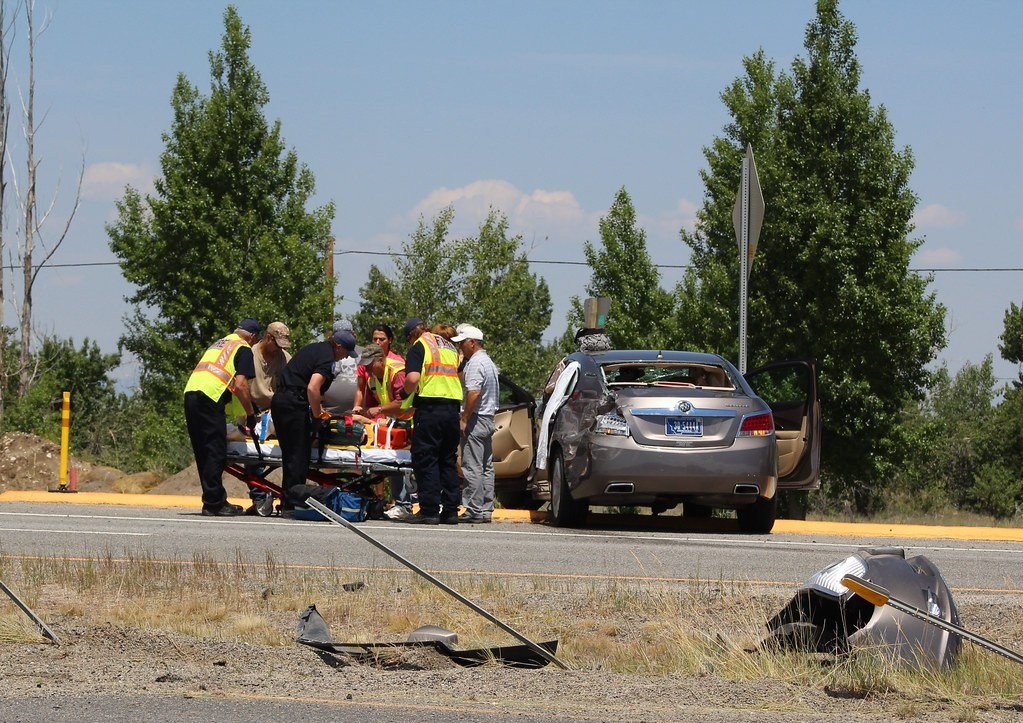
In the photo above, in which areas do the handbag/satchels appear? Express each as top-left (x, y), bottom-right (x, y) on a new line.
top-left (292, 483), bottom-right (369, 522)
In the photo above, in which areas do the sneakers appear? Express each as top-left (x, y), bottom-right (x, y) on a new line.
top-left (456, 513), bottom-right (491, 524)
top-left (438, 512), bottom-right (458, 524)
top-left (383, 504), bottom-right (413, 521)
top-left (403, 508), bottom-right (440, 525)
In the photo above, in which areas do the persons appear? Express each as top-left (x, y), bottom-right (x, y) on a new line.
top-left (618, 369), bottom-right (640, 382)
top-left (183, 317), bottom-right (500, 525)
top-left (690, 368), bottom-right (708, 386)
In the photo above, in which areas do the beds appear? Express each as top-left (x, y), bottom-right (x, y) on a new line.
top-left (226, 441), bottom-right (414, 517)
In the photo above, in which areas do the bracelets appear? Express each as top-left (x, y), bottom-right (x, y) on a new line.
top-left (379, 407), bottom-right (382, 414)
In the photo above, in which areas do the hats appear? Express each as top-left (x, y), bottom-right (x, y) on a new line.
top-left (266, 322), bottom-right (292, 348)
top-left (236, 318), bottom-right (261, 336)
top-left (332, 320), bottom-right (353, 333)
top-left (456, 323), bottom-right (472, 333)
top-left (450, 326), bottom-right (484, 342)
top-left (333, 331), bottom-right (358, 359)
top-left (357, 344), bottom-right (385, 366)
top-left (405, 318), bottom-right (423, 339)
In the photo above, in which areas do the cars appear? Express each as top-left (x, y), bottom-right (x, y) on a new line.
top-left (487, 348), bottom-right (822, 534)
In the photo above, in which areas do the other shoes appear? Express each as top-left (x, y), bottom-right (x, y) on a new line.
top-left (202, 503), bottom-right (243, 516)
